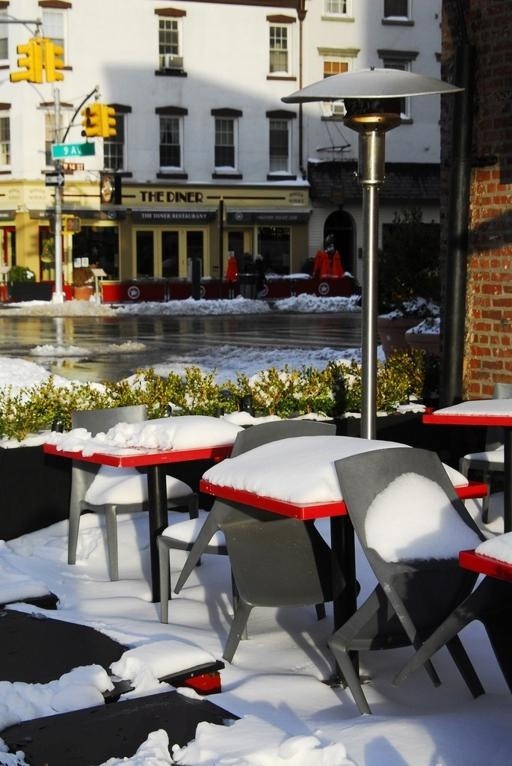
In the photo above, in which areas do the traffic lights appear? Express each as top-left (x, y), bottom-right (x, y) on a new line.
top-left (42, 38), bottom-right (64, 84)
top-left (81, 102), bottom-right (116, 139)
top-left (10, 37), bottom-right (42, 85)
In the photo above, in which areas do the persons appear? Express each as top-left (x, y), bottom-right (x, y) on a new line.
top-left (254, 255), bottom-right (268, 300)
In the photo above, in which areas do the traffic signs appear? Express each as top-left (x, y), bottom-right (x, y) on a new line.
top-left (45, 141), bottom-right (95, 186)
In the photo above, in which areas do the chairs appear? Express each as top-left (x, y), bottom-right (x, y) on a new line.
top-left (156, 419), bottom-right (512, 715)
top-left (460, 382), bottom-right (512, 523)
top-left (68, 406), bottom-right (205, 581)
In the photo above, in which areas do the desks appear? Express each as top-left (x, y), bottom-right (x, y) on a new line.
top-left (0, 591), bottom-right (240, 766)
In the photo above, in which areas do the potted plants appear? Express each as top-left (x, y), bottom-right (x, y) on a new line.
top-left (8, 267), bottom-right (38, 301)
top-left (73, 267), bottom-right (96, 300)
